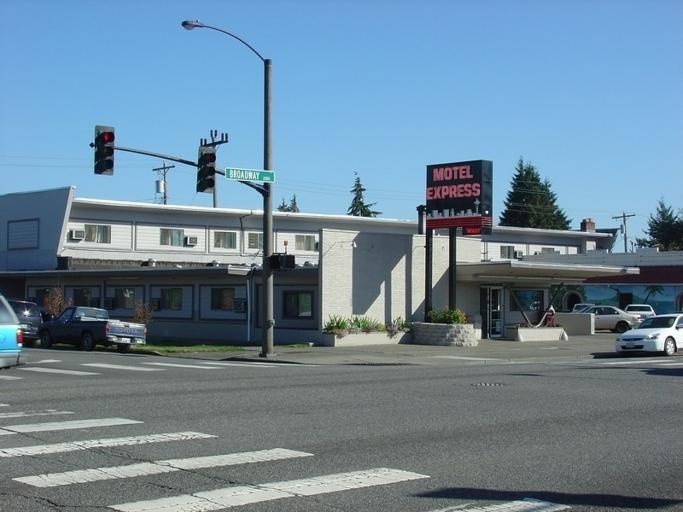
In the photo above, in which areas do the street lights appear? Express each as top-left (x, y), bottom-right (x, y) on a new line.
top-left (178, 19), bottom-right (277, 355)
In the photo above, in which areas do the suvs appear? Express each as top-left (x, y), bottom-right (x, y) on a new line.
top-left (0, 294), bottom-right (144, 368)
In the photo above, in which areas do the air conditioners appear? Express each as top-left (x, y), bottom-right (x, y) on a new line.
top-left (69, 228), bottom-right (85, 240)
top-left (183, 235), bottom-right (197, 246)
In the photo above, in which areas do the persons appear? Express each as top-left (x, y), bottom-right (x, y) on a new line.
top-left (545, 304), bottom-right (556, 327)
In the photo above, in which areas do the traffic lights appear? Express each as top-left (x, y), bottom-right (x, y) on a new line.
top-left (269, 254), bottom-right (294, 269)
top-left (195, 146), bottom-right (215, 195)
top-left (94, 125), bottom-right (113, 176)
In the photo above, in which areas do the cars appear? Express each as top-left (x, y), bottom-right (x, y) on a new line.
top-left (527, 300), bottom-right (539, 310)
top-left (570, 302), bottom-right (656, 333)
top-left (614, 313), bottom-right (682, 355)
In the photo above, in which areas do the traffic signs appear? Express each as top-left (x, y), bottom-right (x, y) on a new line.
top-left (224, 167), bottom-right (275, 184)
